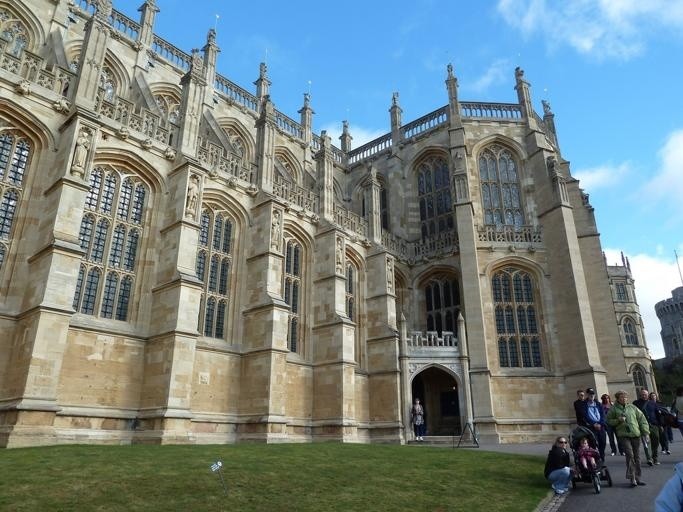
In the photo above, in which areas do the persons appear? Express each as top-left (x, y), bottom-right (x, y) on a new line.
top-left (410, 397), bottom-right (425, 440)
top-left (543, 435), bottom-right (576, 494)
top-left (575, 436), bottom-right (599, 469)
top-left (648, 391), bottom-right (671, 455)
top-left (631, 389), bottom-right (665, 466)
top-left (605, 390), bottom-right (651, 486)
top-left (582, 387), bottom-right (606, 462)
top-left (386, 261), bottom-right (393, 288)
top-left (272, 213), bottom-right (279, 241)
top-left (335, 239), bottom-right (343, 267)
top-left (667, 385), bottom-right (683, 443)
top-left (573, 389), bottom-right (587, 426)
top-left (185, 178), bottom-right (198, 213)
top-left (74, 132), bottom-right (90, 168)
top-left (599, 394), bottom-right (625, 457)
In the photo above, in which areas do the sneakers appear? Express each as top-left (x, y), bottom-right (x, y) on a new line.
top-left (552, 483), bottom-right (569, 495)
top-left (415, 436), bottom-right (423, 441)
top-left (610, 450), bottom-right (672, 487)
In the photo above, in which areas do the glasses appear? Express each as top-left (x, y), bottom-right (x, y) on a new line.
top-left (558, 442), bottom-right (567, 444)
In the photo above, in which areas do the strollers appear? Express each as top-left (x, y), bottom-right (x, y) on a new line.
top-left (567, 426), bottom-right (613, 495)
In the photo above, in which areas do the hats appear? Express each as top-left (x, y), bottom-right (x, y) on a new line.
top-left (586, 388), bottom-right (596, 394)
